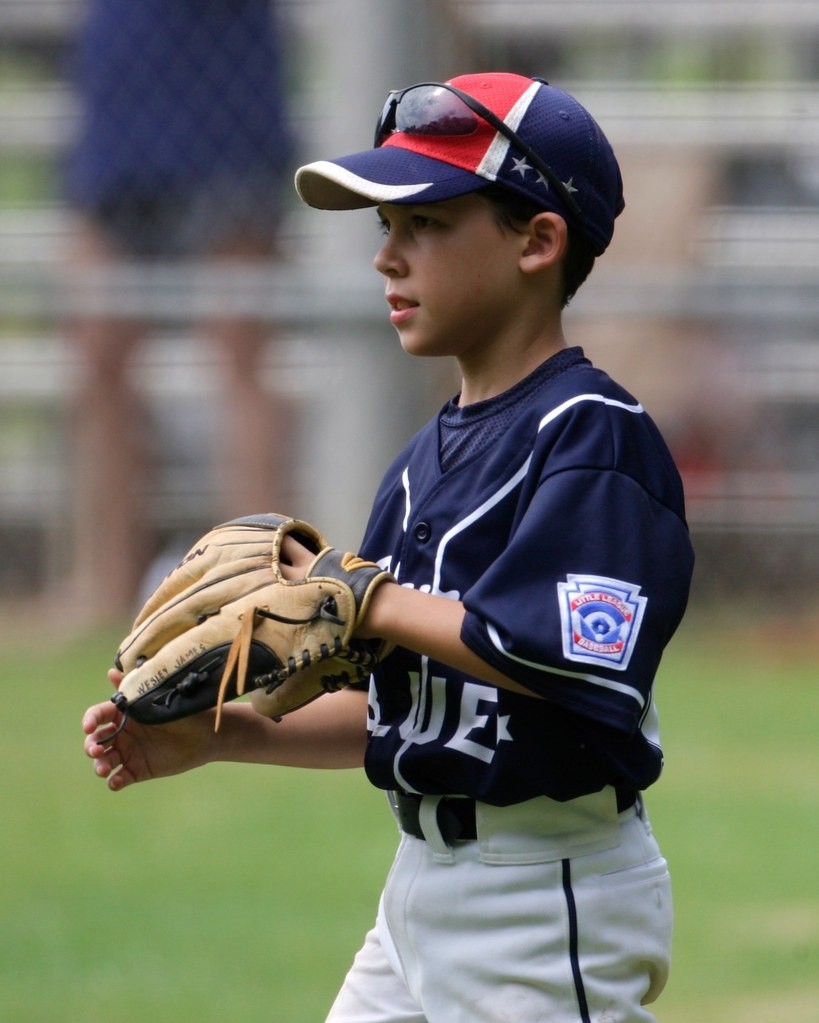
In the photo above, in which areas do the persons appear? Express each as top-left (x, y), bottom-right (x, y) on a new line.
top-left (34, 0), bottom-right (304, 669)
top-left (82, 70), bottom-right (694, 1022)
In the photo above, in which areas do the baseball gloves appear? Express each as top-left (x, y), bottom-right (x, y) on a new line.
top-left (113, 512), bottom-right (399, 729)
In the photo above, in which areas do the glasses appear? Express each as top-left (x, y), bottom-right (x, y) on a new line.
top-left (373, 81), bottom-right (581, 218)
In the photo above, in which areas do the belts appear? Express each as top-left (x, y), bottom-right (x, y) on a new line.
top-left (385, 789), bottom-right (636, 842)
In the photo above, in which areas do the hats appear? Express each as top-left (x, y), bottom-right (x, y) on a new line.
top-left (294, 71), bottom-right (625, 254)
